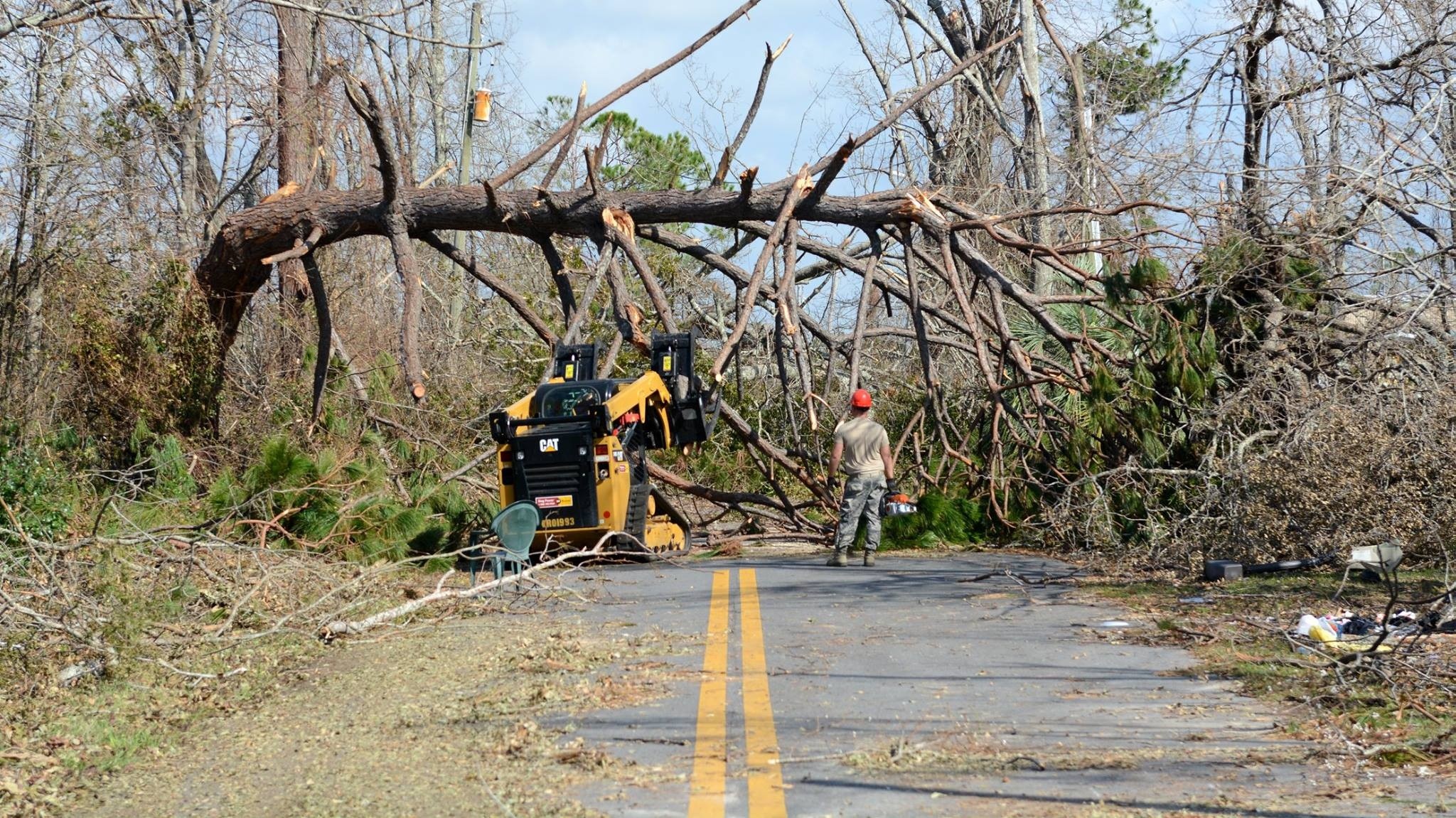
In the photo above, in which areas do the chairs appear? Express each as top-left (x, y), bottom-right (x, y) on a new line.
top-left (469, 499), bottom-right (541, 591)
top-left (1335, 528), bottom-right (1404, 602)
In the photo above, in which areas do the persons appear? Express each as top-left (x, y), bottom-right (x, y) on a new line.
top-left (827, 390), bottom-right (896, 567)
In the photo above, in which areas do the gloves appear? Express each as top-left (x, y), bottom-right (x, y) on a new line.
top-left (886, 479), bottom-right (901, 495)
top-left (828, 477), bottom-right (838, 492)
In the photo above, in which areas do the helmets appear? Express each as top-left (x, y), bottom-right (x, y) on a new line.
top-left (850, 389), bottom-right (871, 407)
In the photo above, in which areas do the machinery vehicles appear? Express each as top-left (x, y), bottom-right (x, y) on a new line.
top-left (490, 332), bottom-right (722, 564)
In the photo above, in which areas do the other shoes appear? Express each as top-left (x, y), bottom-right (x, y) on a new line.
top-left (865, 556), bottom-right (875, 566)
top-left (827, 553), bottom-right (847, 566)
